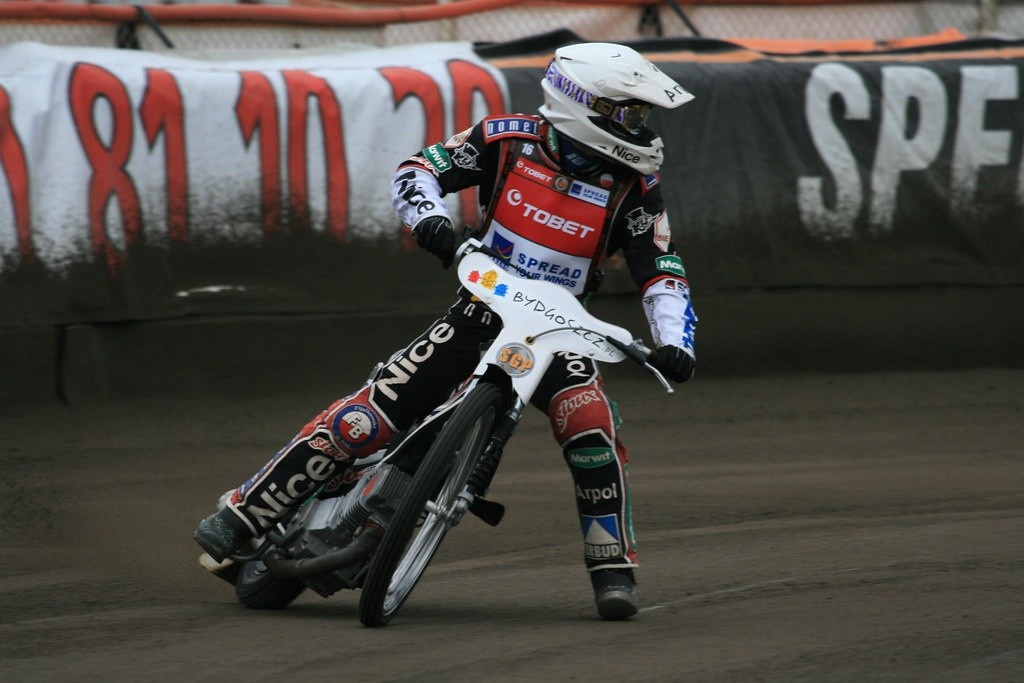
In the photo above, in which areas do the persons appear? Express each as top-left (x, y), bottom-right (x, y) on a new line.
top-left (192, 43), bottom-right (700, 619)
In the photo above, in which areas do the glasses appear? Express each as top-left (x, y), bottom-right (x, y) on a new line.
top-left (593, 98), bottom-right (657, 132)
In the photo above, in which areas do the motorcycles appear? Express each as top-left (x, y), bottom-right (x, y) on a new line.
top-left (199, 223), bottom-right (697, 629)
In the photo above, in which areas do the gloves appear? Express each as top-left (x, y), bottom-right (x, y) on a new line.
top-left (658, 346), bottom-right (692, 384)
top-left (415, 218), bottom-right (455, 268)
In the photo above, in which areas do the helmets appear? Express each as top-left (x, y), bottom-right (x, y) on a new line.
top-left (538, 42), bottom-right (696, 177)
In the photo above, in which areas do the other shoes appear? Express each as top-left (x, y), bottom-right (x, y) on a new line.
top-left (194, 512), bottom-right (231, 564)
top-left (595, 585), bottom-right (637, 621)
